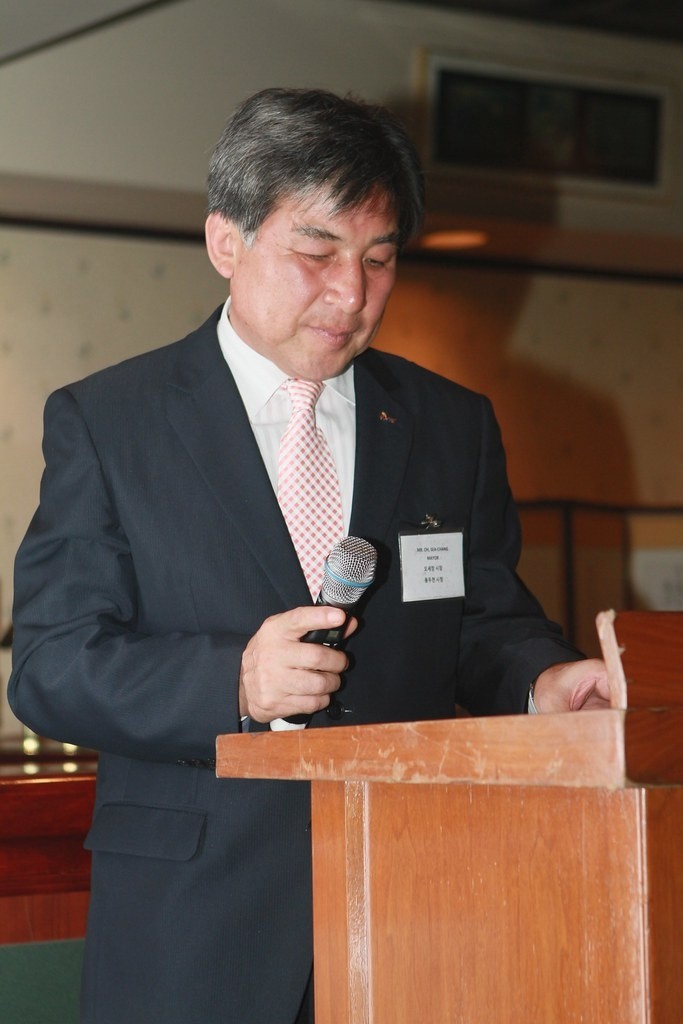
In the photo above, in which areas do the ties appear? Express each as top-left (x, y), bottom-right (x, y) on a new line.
top-left (276, 378), bottom-right (346, 605)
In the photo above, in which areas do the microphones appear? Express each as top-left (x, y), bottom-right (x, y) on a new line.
top-left (270, 536), bottom-right (378, 732)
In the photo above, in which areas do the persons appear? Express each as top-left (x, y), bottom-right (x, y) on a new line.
top-left (8, 86), bottom-right (610, 1024)
